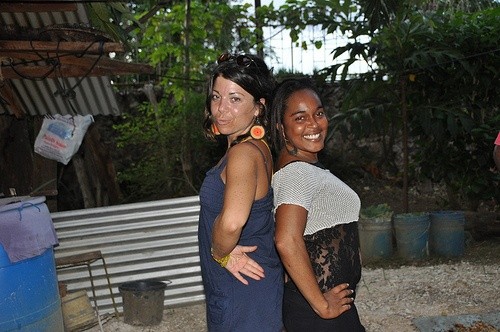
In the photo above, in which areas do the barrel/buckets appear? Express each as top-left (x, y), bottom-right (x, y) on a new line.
top-left (60, 290), bottom-right (99, 332)
top-left (393, 212), bottom-right (431, 267)
top-left (358, 216), bottom-right (393, 268)
top-left (118, 280), bottom-right (167, 326)
top-left (430, 210), bottom-right (465, 265)
top-left (0, 195), bottom-right (64, 332)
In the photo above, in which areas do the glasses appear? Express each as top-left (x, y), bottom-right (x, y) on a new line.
top-left (218, 53), bottom-right (260, 70)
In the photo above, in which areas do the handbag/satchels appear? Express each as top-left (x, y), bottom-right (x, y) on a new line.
top-left (34, 114), bottom-right (95, 166)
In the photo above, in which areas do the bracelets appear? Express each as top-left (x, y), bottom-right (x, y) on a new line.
top-left (210, 242), bottom-right (231, 268)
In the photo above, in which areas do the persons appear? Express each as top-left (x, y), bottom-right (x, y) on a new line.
top-left (198, 53), bottom-right (284, 332)
top-left (269, 79), bottom-right (365, 332)
